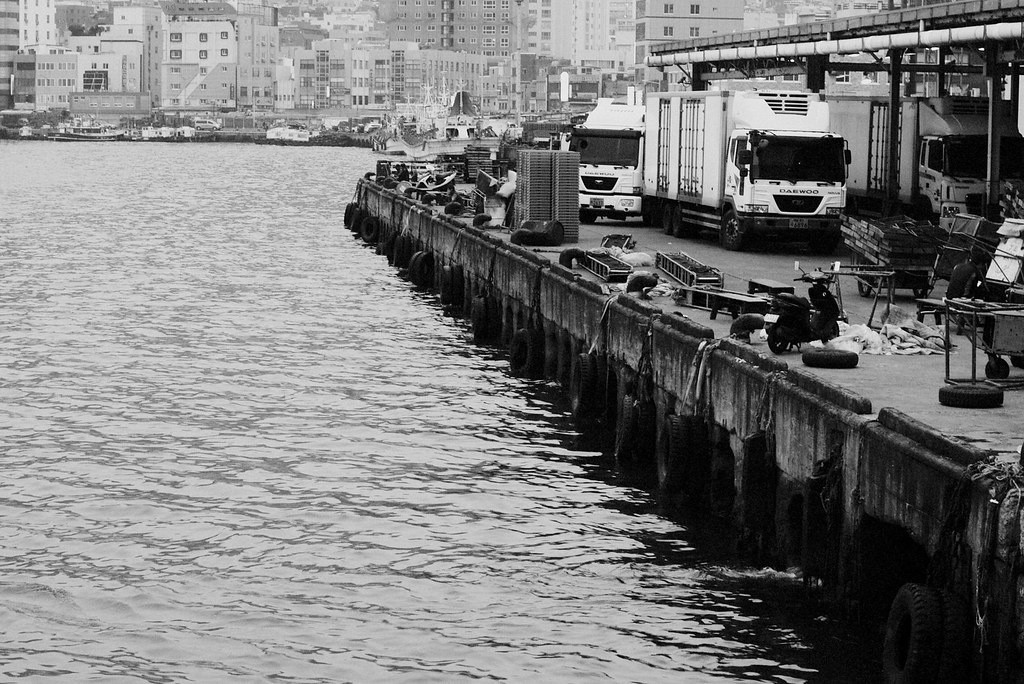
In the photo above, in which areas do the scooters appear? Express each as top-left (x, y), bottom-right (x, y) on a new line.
top-left (762, 261), bottom-right (843, 355)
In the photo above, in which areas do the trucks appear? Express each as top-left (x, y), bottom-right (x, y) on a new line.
top-left (640, 87), bottom-right (852, 253)
top-left (828, 93), bottom-right (1024, 220)
top-left (562, 104), bottom-right (646, 224)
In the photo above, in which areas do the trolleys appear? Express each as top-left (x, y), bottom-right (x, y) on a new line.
top-left (917, 297), bottom-right (1024, 380)
top-left (849, 246), bottom-right (936, 305)
top-left (929, 210), bottom-right (1024, 297)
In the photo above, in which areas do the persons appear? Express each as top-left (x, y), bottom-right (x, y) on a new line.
top-left (394, 164), bottom-right (464, 206)
top-left (945, 251), bottom-right (992, 299)
